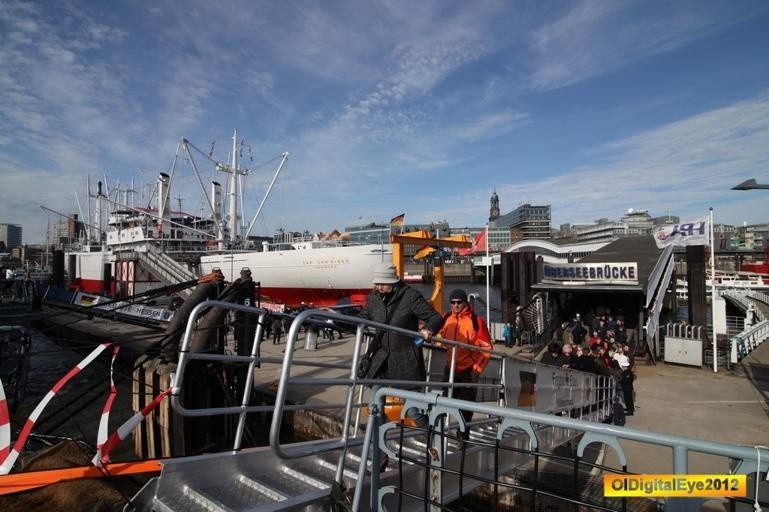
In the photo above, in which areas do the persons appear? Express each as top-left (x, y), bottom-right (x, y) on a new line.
top-left (321, 262), bottom-right (445, 476)
top-left (264, 302), bottom-right (343, 345)
top-left (534, 303), bottom-right (637, 424)
top-left (502, 315), bottom-right (524, 349)
top-left (5, 265), bottom-right (15, 279)
top-left (422, 288), bottom-right (494, 450)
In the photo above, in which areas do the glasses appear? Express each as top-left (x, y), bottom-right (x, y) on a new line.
top-left (450, 299), bottom-right (465, 304)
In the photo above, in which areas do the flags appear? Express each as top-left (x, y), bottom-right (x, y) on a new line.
top-left (390, 213), bottom-right (404, 228)
top-left (653, 213), bottom-right (709, 249)
top-left (458, 228), bottom-right (486, 255)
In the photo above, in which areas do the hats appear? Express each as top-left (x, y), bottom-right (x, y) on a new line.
top-left (449, 287), bottom-right (467, 303)
top-left (373, 262), bottom-right (401, 286)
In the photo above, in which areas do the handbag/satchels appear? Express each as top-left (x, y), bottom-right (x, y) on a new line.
top-left (357, 352), bottom-right (371, 378)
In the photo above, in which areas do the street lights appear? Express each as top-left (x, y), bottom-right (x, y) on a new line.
top-left (731, 178), bottom-right (769, 190)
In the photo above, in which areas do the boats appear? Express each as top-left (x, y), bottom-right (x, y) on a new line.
top-left (25, 127), bottom-right (393, 330)
top-left (675, 261), bottom-right (769, 302)
